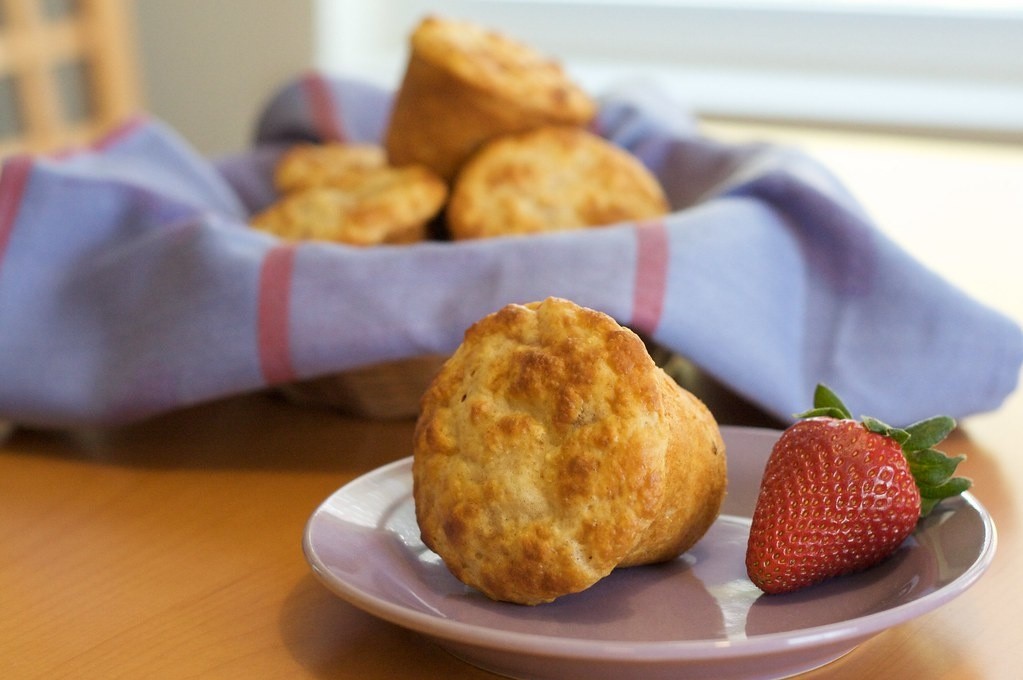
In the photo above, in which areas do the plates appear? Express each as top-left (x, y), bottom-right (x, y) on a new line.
top-left (301, 425), bottom-right (998, 680)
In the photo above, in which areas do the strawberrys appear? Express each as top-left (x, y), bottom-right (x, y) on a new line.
top-left (745, 384), bottom-right (974, 592)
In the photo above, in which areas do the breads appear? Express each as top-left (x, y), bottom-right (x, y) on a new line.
top-left (247, 9), bottom-right (669, 244)
top-left (410, 299), bottom-right (729, 606)
